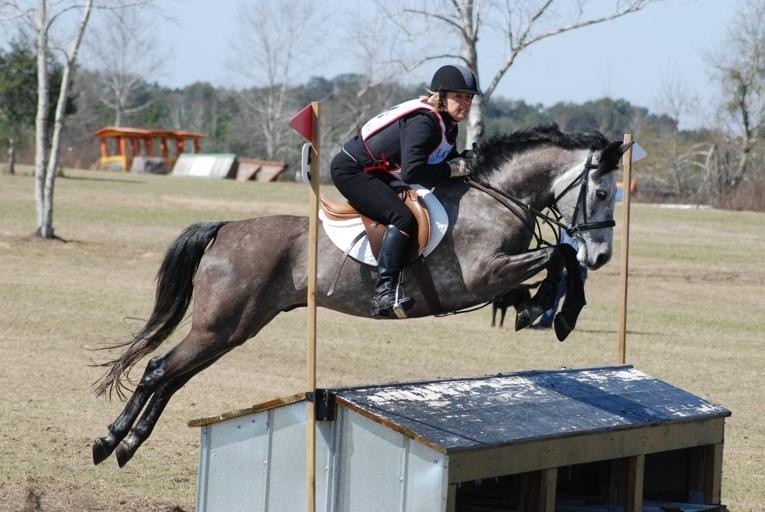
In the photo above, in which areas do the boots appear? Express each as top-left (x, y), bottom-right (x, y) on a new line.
top-left (371, 225), bottom-right (415, 316)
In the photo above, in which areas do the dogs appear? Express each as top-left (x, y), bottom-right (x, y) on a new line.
top-left (489, 279), bottom-right (544, 331)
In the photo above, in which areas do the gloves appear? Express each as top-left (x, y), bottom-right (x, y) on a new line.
top-left (446, 157), bottom-right (470, 177)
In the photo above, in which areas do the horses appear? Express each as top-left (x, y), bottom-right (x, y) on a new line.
top-left (85, 120), bottom-right (637, 469)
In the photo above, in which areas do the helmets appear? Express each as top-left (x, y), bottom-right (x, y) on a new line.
top-left (431, 66), bottom-right (479, 95)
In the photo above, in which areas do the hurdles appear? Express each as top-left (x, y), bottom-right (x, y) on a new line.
top-left (186, 101), bottom-right (732, 512)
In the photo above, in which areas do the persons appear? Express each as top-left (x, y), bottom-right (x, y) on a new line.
top-left (330, 64), bottom-right (484, 318)
